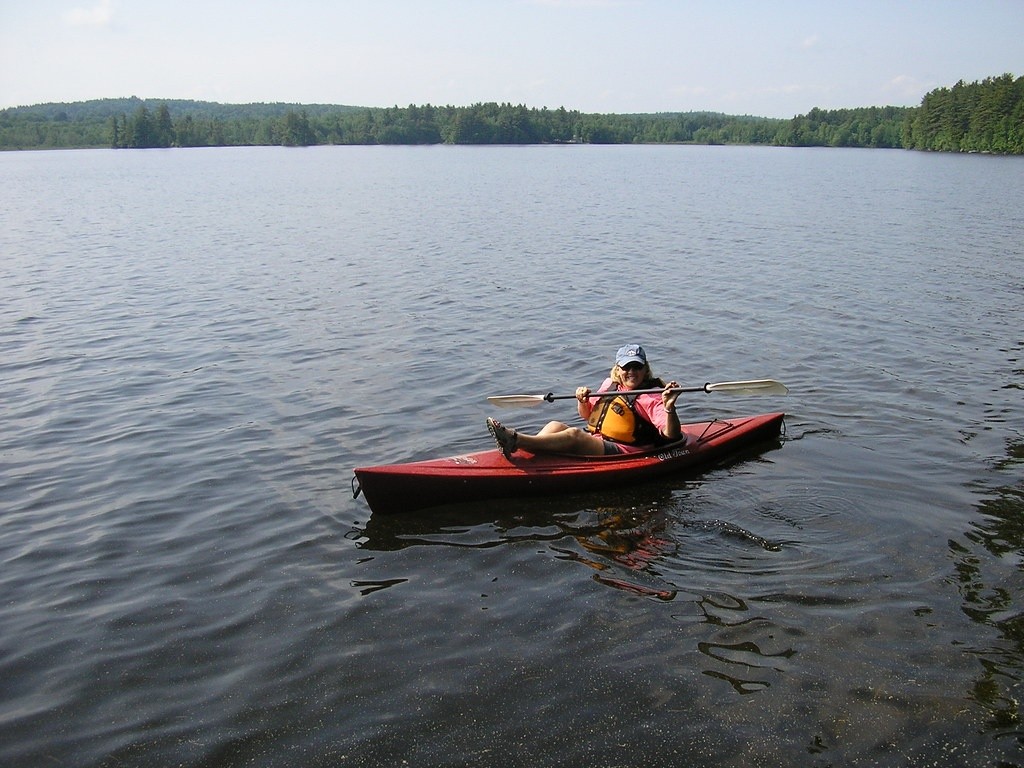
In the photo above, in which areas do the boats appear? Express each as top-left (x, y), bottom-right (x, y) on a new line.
top-left (350, 409), bottom-right (786, 517)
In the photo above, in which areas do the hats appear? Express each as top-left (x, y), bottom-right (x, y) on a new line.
top-left (616, 344), bottom-right (646, 367)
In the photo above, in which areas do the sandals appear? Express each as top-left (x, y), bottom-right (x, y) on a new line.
top-left (486, 417), bottom-right (518, 459)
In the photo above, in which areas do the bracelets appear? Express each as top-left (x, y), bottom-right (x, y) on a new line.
top-left (664, 407), bottom-right (676, 414)
top-left (577, 398), bottom-right (590, 404)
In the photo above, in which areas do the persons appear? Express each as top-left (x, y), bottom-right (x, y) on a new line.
top-left (486, 344), bottom-right (683, 460)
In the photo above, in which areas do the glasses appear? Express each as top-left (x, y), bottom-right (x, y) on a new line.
top-left (618, 362), bottom-right (645, 371)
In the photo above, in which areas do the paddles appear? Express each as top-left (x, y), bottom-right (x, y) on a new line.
top-left (486, 379), bottom-right (793, 408)
